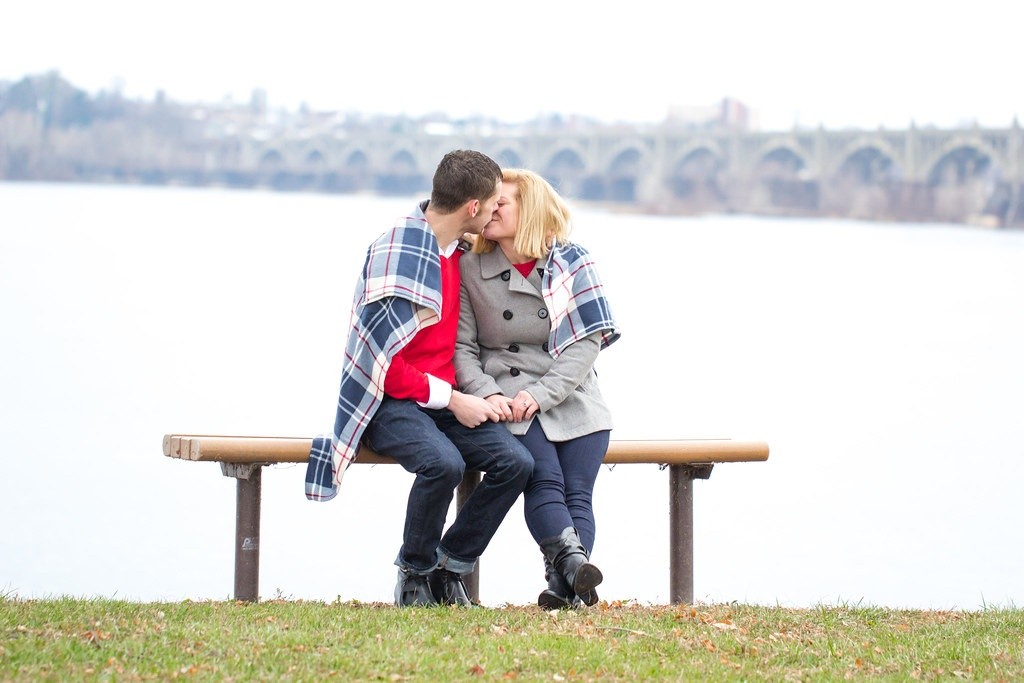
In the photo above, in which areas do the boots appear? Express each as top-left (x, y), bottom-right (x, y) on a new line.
top-left (540, 527), bottom-right (602, 607)
top-left (538, 550), bottom-right (591, 611)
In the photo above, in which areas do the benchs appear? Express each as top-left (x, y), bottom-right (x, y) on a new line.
top-left (162, 434), bottom-right (768, 606)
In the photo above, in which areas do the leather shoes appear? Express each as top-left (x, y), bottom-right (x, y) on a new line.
top-left (432, 569), bottom-right (477, 607)
top-left (394, 570), bottom-right (438, 607)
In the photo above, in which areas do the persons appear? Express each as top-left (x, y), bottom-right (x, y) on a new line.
top-left (453, 169), bottom-right (622, 609)
top-left (305, 148), bottom-right (534, 609)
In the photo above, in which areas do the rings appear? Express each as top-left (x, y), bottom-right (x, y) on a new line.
top-left (523, 401), bottom-right (528, 408)
